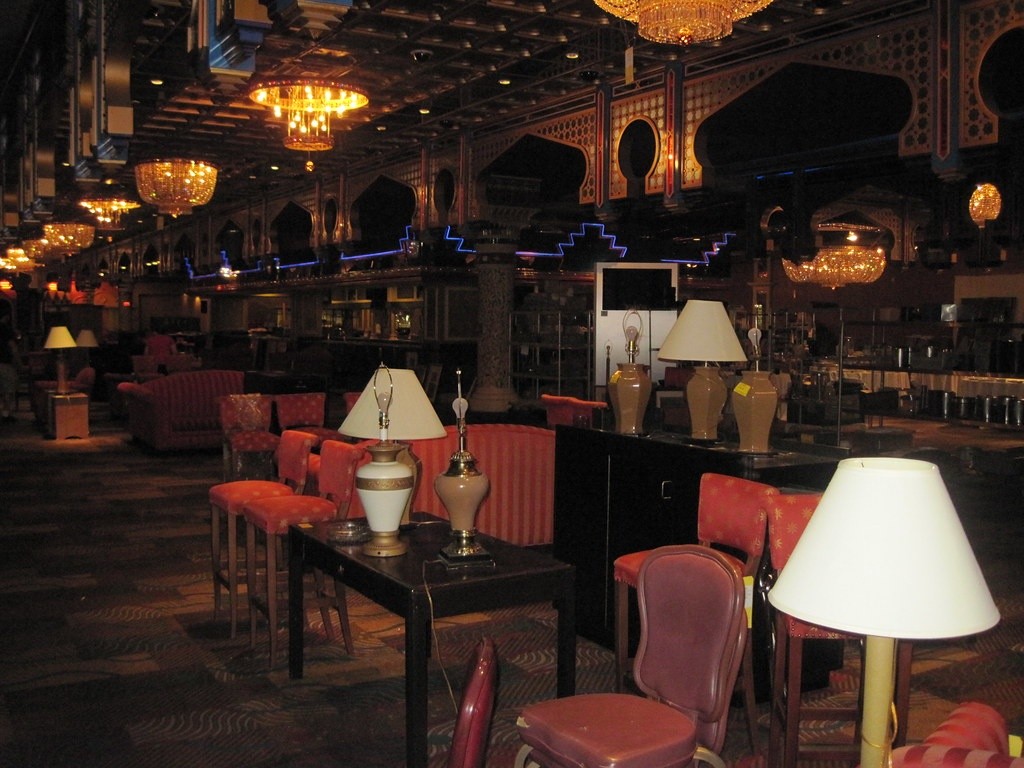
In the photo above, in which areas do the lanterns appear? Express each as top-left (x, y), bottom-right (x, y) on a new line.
top-left (969, 183), bottom-right (1002, 232)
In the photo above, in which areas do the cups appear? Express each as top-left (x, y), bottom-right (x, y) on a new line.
top-left (891, 345), bottom-right (912, 370)
top-left (940, 391), bottom-right (1024, 428)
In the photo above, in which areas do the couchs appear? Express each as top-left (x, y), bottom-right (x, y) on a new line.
top-left (319, 422), bottom-right (554, 548)
top-left (120, 369), bottom-right (260, 450)
top-left (131, 356), bottom-right (160, 373)
top-left (892, 694), bottom-right (1024, 768)
top-left (145, 336), bottom-right (176, 357)
top-left (539, 393), bottom-right (609, 428)
top-left (104, 369), bottom-right (165, 424)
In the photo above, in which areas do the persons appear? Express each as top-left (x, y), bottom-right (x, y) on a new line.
top-left (146, 327), bottom-right (177, 359)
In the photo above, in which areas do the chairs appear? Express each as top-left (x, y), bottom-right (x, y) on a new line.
top-left (210, 391), bottom-right (917, 768)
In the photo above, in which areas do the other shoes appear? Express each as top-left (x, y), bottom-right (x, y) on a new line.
top-left (1, 415), bottom-right (17, 424)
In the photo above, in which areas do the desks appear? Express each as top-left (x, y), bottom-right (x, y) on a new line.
top-left (842, 368), bottom-right (1024, 429)
top-left (247, 367), bottom-right (314, 391)
top-left (281, 512), bottom-right (581, 768)
top-left (132, 353), bottom-right (203, 370)
top-left (47, 393), bottom-right (90, 439)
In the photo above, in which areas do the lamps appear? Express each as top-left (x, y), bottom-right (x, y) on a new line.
top-left (44, 326), bottom-right (77, 394)
top-left (613, 298), bottom-right (779, 454)
top-left (336, 365), bottom-right (493, 554)
top-left (0, 0), bottom-right (1002, 294)
top-left (768, 454), bottom-right (1000, 768)
top-left (78, 328), bottom-right (99, 384)
